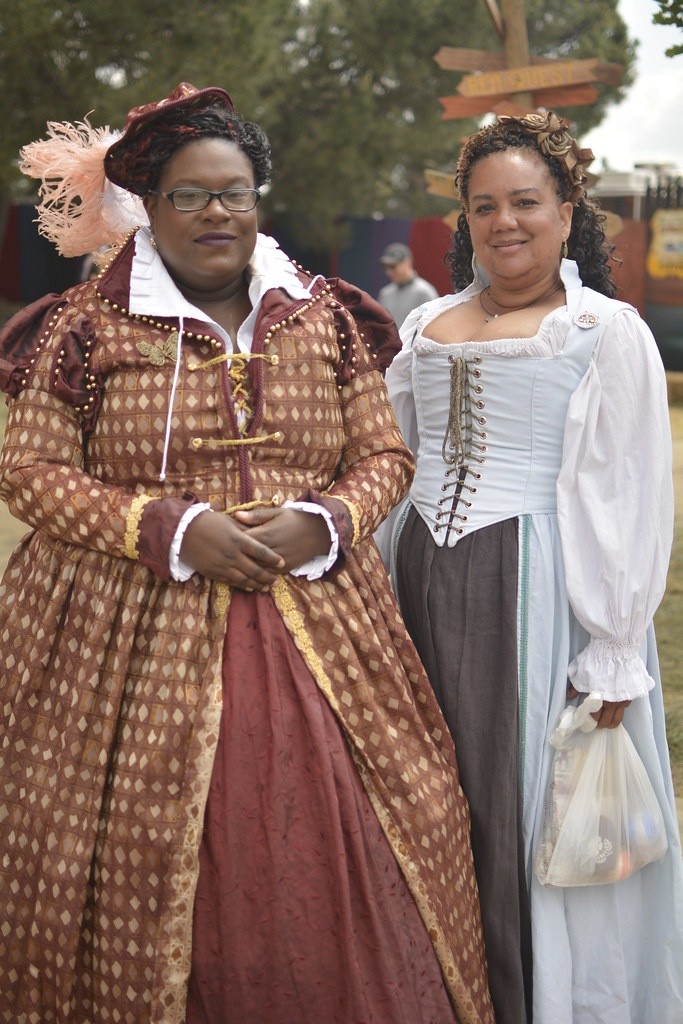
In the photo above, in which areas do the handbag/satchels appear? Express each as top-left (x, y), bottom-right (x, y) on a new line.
top-left (536, 699), bottom-right (668, 888)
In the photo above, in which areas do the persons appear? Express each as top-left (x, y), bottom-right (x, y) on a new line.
top-left (0, 80), bottom-right (494, 1024)
top-left (372, 114), bottom-right (683, 1024)
top-left (378, 242), bottom-right (439, 331)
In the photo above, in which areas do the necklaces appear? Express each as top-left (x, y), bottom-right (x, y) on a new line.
top-left (480, 285), bottom-right (563, 322)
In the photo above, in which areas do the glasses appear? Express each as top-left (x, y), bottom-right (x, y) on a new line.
top-left (148, 188), bottom-right (261, 212)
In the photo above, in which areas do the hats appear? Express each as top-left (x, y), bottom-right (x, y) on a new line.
top-left (379, 243), bottom-right (412, 266)
